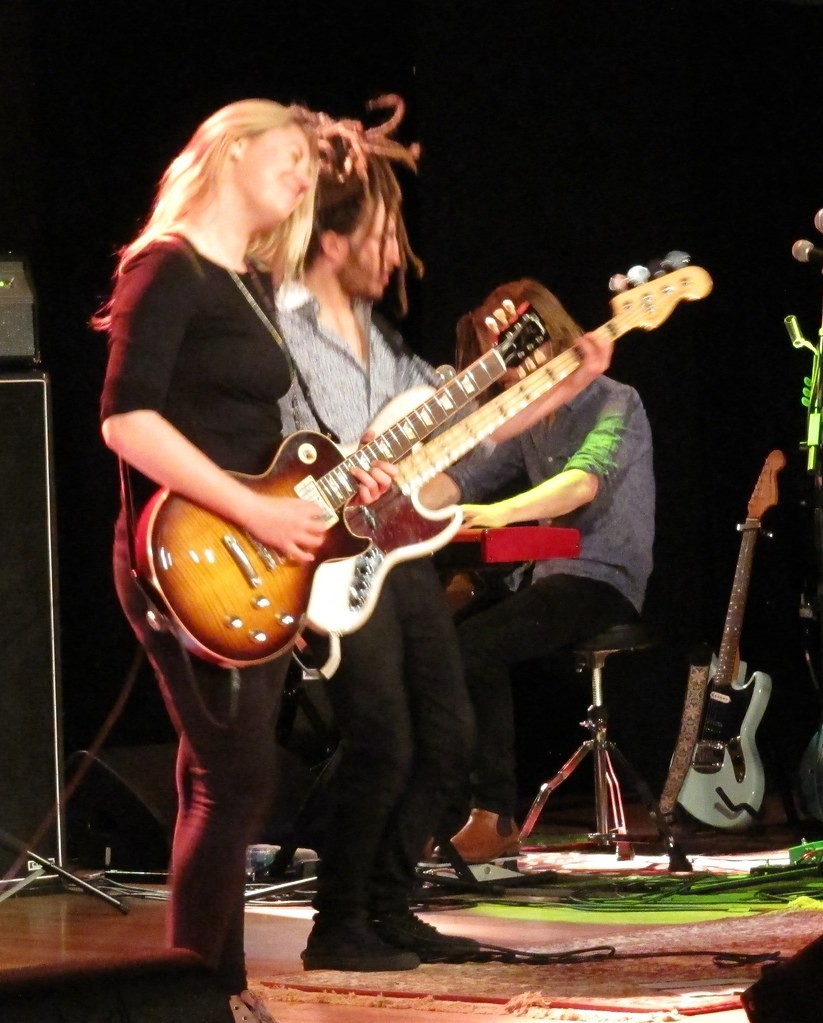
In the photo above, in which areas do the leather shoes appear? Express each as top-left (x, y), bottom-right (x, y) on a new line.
top-left (432, 808), bottom-right (520, 865)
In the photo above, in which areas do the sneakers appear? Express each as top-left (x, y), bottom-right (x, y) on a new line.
top-left (300, 915), bottom-right (422, 971)
top-left (375, 910), bottom-right (481, 963)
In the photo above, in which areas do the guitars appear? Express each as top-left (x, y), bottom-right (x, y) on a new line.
top-left (668, 449), bottom-right (787, 831)
top-left (305, 250), bottom-right (713, 636)
top-left (135, 299), bottom-right (554, 669)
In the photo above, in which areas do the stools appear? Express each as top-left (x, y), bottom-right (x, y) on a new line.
top-left (518, 614), bottom-right (694, 875)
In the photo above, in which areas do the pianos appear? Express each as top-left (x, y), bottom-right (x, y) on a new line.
top-left (433, 526), bottom-right (580, 572)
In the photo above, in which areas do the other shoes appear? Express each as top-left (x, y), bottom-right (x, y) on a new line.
top-left (227, 991), bottom-right (277, 1023)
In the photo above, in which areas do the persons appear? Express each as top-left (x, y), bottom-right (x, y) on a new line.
top-left (418, 280), bottom-right (656, 864)
top-left (100, 98), bottom-right (398, 1023)
top-left (272, 147), bottom-right (615, 972)
